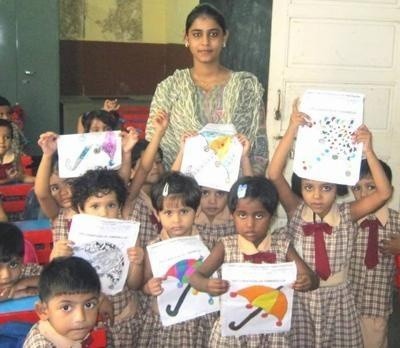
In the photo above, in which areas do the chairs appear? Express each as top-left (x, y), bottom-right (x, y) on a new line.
top-left (0, 106), bottom-right (151, 348)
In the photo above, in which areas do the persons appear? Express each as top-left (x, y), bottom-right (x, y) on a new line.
top-left (143, 4), bottom-right (269, 180)
top-left (1, 94), bottom-right (400, 348)
